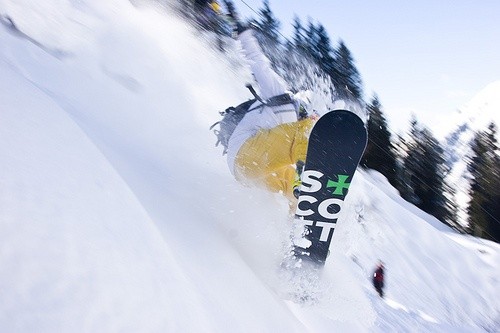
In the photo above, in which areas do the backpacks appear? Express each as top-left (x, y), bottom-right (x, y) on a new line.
top-left (209, 85), bottom-right (292, 156)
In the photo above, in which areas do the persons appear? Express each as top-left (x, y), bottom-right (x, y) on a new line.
top-left (210, 23), bottom-right (319, 220)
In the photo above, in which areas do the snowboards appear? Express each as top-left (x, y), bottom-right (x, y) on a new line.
top-left (281, 110), bottom-right (368, 271)
